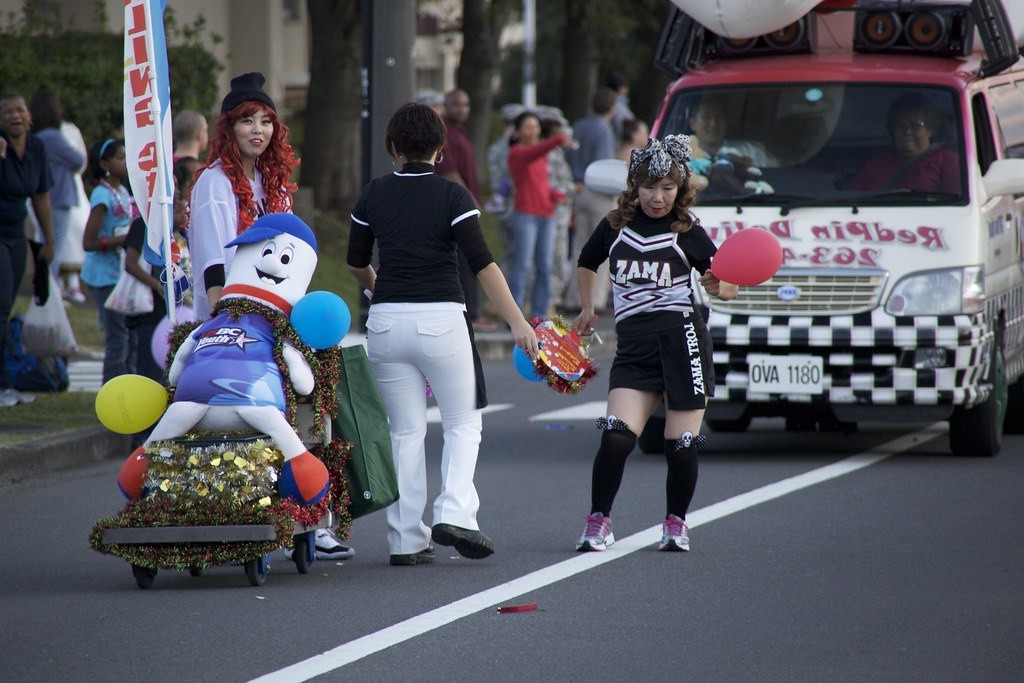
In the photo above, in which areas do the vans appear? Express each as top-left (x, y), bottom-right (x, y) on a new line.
top-left (584, 51), bottom-right (1024, 459)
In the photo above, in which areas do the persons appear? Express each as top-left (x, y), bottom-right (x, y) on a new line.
top-left (841, 91), bottom-right (962, 196)
top-left (81, 138), bottom-right (133, 386)
top-left (433, 72), bottom-right (650, 332)
top-left (28, 86), bottom-right (91, 303)
top-left (187, 71), bottom-right (356, 559)
top-left (120, 173), bottom-right (181, 454)
top-left (686, 96), bottom-right (731, 194)
top-left (346, 103), bottom-right (540, 566)
top-left (172, 109), bottom-right (209, 201)
top-left (569, 133), bottom-right (741, 552)
top-left (0, 92), bottom-right (55, 406)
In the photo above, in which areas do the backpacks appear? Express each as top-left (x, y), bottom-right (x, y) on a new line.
top-left (1, 315), bottom-right (69, 393)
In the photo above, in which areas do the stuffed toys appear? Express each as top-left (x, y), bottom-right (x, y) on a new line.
top-left (116, 213), bottom-right (330, 504)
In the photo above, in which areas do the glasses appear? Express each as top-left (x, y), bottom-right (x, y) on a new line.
top-left (895, 122), bottom-right (924, 131)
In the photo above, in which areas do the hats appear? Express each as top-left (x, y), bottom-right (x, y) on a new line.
top-left (222, 71), bottom-right (277, 111)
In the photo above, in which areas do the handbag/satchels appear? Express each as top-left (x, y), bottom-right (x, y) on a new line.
top-left (22, 268), bottom-right (78, 356)
top-left (104, 246), bottom-right (155, 315)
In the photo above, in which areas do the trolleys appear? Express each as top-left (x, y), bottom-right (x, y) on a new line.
top-left (99, 405), bottom-right (332, 590)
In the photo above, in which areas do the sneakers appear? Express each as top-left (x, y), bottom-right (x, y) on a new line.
top-left (391, 548), bottom-right (435, 564)
top-left (284, 527), bottom-right (355, 560)
top-left (431, 523), bottom-right (494, 560)
top-left (659, 515), bottom-right (690, 551)
top-left (576, 513), bottom-right (615, 551)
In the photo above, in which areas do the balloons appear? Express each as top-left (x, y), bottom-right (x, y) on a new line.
top-left (290, 290), bottom-right (351, 350)
top-left (710, 228), bottom-right (783, 287)
top-left (150, 304), bottom-right (194, 371)
top-left (95, 374), bottom-right (168, 435)
top-left (512, 343), bottom-right (548, 382)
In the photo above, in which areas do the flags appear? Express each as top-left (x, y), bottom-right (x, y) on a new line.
top-left (124, 0), bottom-right (175, 268)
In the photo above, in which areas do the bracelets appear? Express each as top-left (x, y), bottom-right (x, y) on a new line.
top-left (716, 290), bottom-right (722, 297)
top-left (101, 236), bottom-right (107, 250)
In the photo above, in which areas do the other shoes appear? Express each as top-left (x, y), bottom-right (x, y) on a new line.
top-left (65, 287), bottom-right (87, 305)
top-left (0, 388), bottom-right (19, 407)
top-left (472, 319), bottom-right (497, 333)
top-left (19, 391), bottom-right (37, 403)
